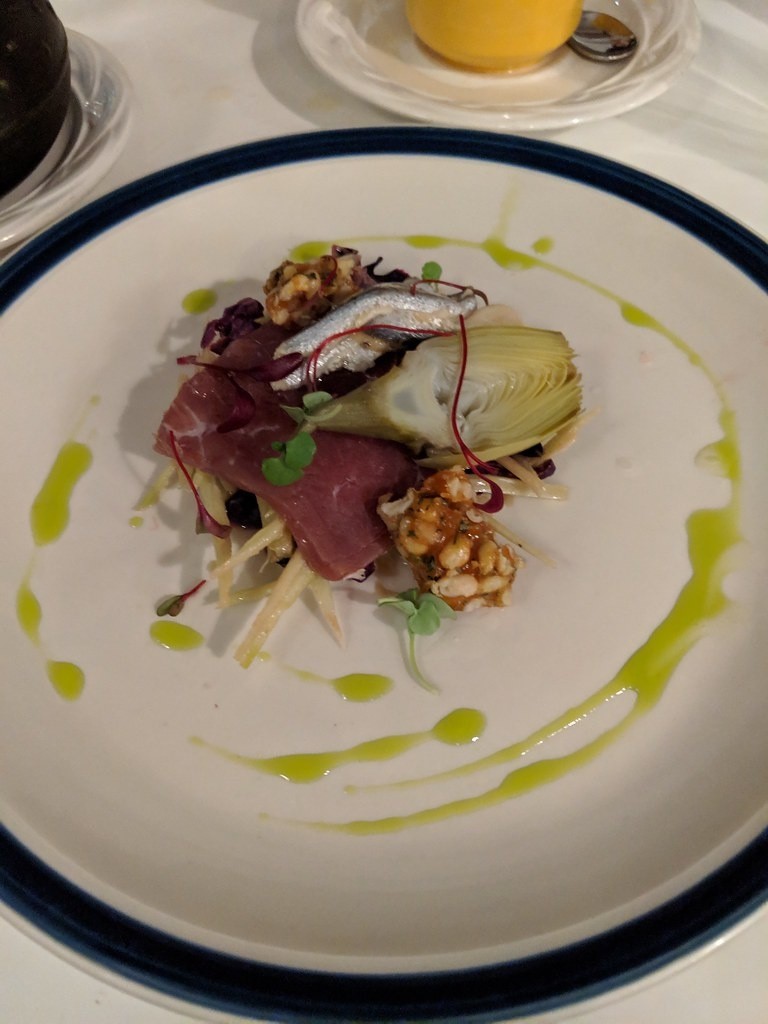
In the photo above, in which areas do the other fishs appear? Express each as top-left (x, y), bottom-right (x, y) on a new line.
top-left (270, 281), bottom-right (478, 393)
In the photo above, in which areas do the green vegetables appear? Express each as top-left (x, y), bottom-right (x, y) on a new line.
top-left (261, 388), bottom-right (456, 695)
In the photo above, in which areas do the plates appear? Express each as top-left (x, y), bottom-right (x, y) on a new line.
top-left (0, 25), bottom-right (135, 253)
top-left (0, 123), bottom-right (768, 1024)
top-left (296, 0), bottom-right (703, 134)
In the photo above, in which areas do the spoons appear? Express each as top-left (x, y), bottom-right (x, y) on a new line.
top-left (566, 10), bottom-right (638, 62)
